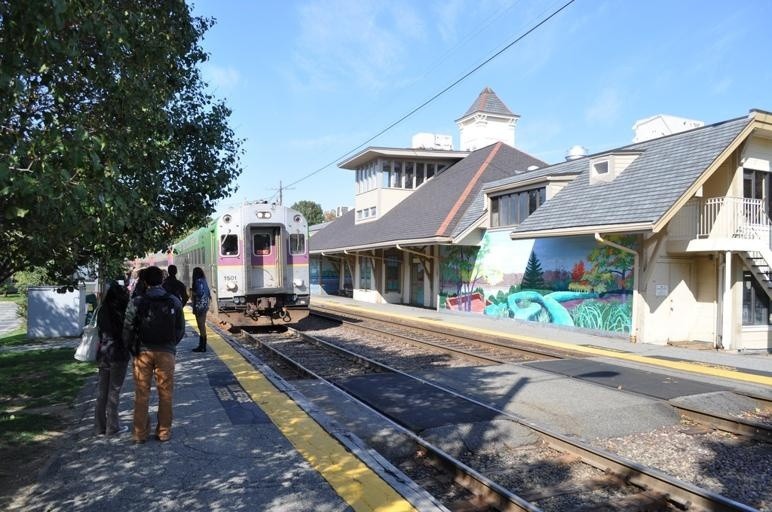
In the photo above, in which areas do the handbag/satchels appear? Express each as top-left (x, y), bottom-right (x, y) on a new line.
top-left (74, 324), bottom-right (100, 362)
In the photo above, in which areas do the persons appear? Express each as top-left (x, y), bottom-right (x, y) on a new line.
top-left (191, 266), bottom-right (211, 354)
top-left (133, 268), bottom-right (148, 297)
top-left (93, 286), bottom-right (132, 437)
top-left (164, 262), bottom-right (189, 306)
top-left (122, 265), bottom-right (187, 445)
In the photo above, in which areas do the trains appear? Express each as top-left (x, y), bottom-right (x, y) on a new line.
top-left (130, 202), bottom-right (312, 331)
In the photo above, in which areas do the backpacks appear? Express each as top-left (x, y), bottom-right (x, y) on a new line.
top-left (135, 296), bottom-right (177, 344)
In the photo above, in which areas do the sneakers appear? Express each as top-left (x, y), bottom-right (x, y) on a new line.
top-left (160, 437), bottom-right (169, 440)
top-left (135, 440), bottom-right (145, 445)
top-left (98, 426), bottom-right (128, 437)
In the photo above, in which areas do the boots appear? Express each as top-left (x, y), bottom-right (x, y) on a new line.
top-left (192, 335), bottom-right (206, 352)
